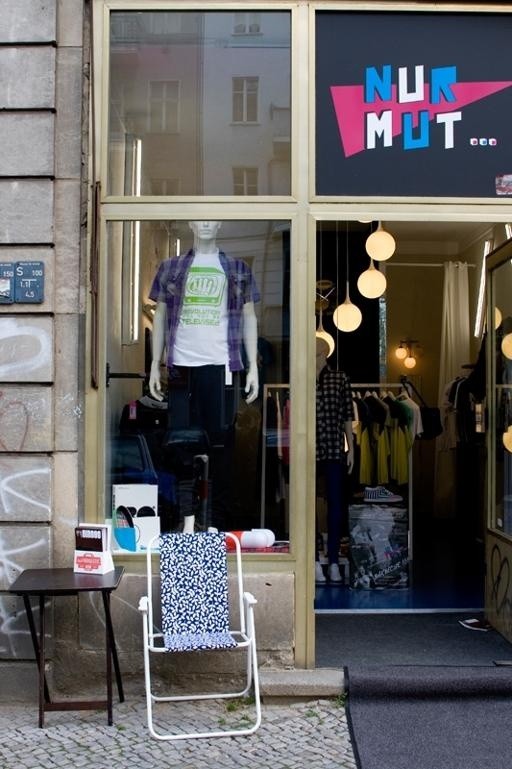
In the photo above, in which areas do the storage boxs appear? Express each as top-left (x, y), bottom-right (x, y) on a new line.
top-left (348, 501), bottom-right (410, 591)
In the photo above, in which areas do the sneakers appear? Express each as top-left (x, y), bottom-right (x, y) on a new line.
top-left (457, 617), bottom-right (490, 633)
top-left (363, 485), bottom-right (404, 503)
top-left (112, 504), bottom-right (138, 553)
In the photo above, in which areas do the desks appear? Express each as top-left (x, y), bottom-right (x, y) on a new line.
top-left (8, 566), bottom-right (126, 725)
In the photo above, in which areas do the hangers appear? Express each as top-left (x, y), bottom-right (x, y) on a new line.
top-left (266, 384), bottom-right (414, 405)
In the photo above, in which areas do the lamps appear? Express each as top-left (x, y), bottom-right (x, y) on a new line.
top-left (315, 220), bottom-right (396, 362)
top-left (116, 130), bottom-right (146, 349)
top-left (494, 306), bottom-right (512, 363)
top-left (168, 234), bottom-right (182, 259)
top-left (396, 339), bottom-right (421, 369)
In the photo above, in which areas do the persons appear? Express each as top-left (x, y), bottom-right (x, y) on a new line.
top-left (316, 338), bottom-right (356, 582)
top-left (148, 220), bottom-right (260, 534)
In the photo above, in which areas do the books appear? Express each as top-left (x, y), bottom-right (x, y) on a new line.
top-left (79, 523), bottom-right (111, 552)
top-left (75, 526), bottom-right (107, 552)
top-left (458, 617), bottom-right (493, 632)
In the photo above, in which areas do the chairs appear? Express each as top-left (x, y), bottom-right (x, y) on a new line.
top-left (139, 531), bottom-right (266, 739)
